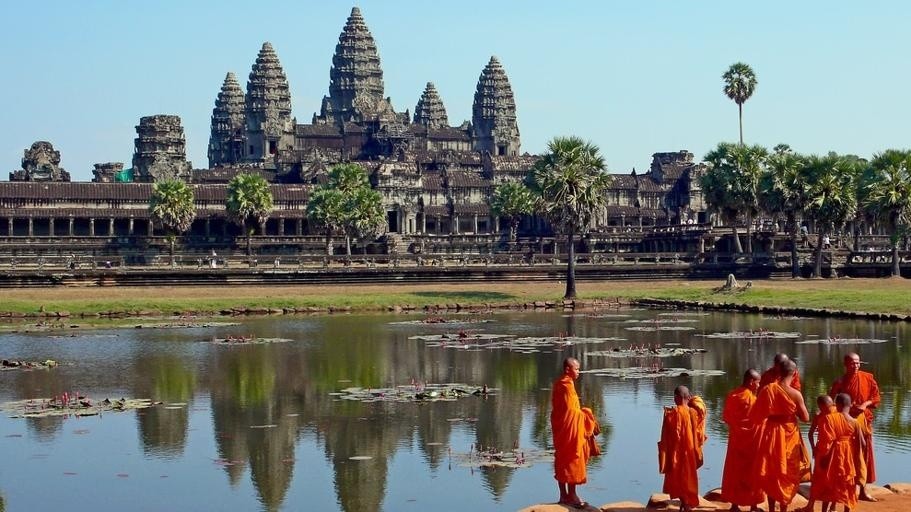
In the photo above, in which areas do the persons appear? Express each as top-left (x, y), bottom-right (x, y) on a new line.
top-left (802, 231), bottom-right (847, 249)
top-left (657, 352), bottom-right (881, 512)
top-left (551, 358), bottom-right (599, 508)
